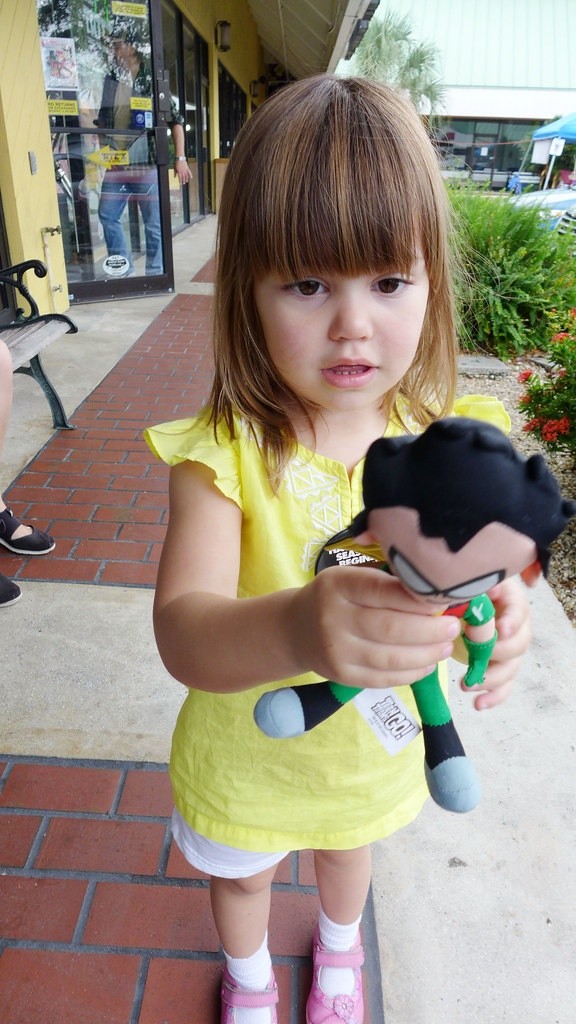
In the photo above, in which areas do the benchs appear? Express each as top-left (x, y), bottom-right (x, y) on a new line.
top-left (0, 260), bottom-right (78, 431)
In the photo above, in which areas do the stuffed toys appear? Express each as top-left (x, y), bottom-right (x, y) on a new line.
top-left (255, 417), bottom-right (575, 813)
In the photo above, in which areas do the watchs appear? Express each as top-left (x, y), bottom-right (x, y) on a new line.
top-left (174, 156), bottom-right (186, 162)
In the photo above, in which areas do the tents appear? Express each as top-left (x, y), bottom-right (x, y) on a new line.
top-left (508, 111), bottom-right (576, 195)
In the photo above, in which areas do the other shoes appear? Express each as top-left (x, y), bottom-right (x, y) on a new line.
top-left (0, 573), bottom-right (22, 608)
top-left (220, 961), bottom-right (279, 1024)
top-left (306, 923), bottom-right (365, 1024)
top-left (0, 507), bottom-right (55, 555)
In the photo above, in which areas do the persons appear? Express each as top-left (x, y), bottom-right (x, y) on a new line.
top-left (0, 339), bottom-right (56, 608)
top-left (93, 30), bottom-right (193, 277)
top-left (141, 75), bottom-right (532, 1024)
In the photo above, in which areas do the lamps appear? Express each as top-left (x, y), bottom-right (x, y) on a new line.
top-left (249, 80), bottom-right (260, 97)
top-left (214, 19), bottom-right (232, 51)
